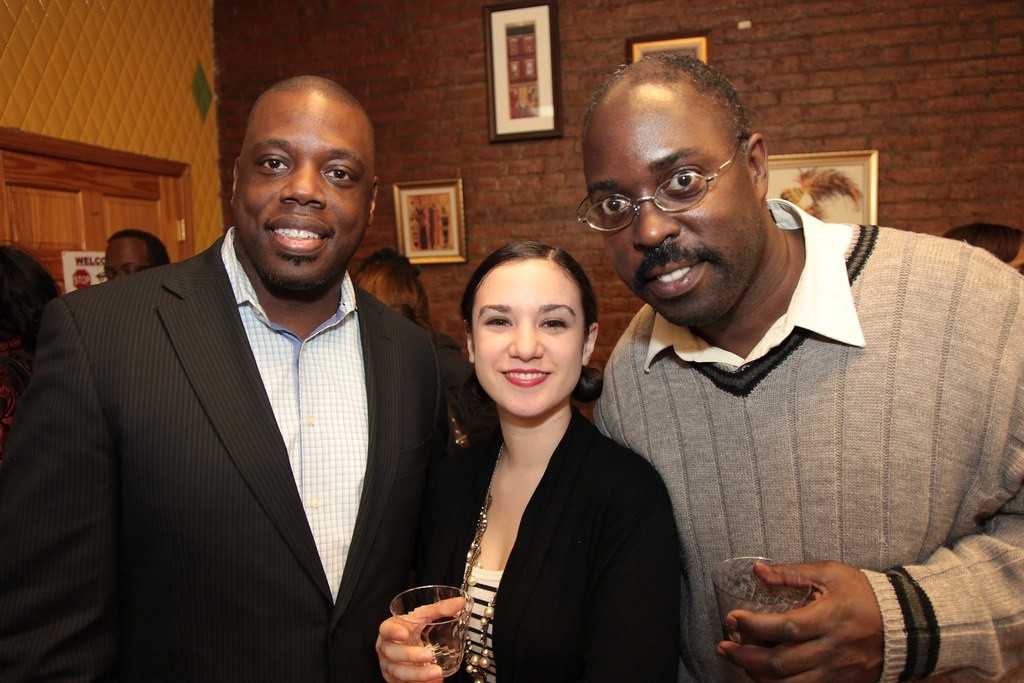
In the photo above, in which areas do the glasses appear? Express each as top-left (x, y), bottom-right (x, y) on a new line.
top-left (576, 130), bottom-right (750, 231)
top-left (97, 267), bottom-right (143, 284)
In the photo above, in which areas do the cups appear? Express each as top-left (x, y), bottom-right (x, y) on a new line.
top-left (711, 556), bottom-right (811, 648)
top-left (390, 585), bottom-right (474, 678)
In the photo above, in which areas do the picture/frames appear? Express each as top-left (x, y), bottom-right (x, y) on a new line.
top-left (763, 148), bottom-right (878, 227)
top-left (625, 29), bottom-right (711, 71)
top-left (483, 1), bottom-right (565, 142)
top-left (392, 178), bottom-right (466, 264)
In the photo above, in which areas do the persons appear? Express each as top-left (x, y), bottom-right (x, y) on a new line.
top-left (0, 245), bottom-right (62, 467)
top-left (583, 54), bottom-right (1023, 683)
top-left (104, 229), bottom-right (170, 281)
top-left (0, 75), bottom-right (447, 683)
top-left (375, 241), bottom-right (684, 683)
top-left (354, 248), bottom-right (470, 446)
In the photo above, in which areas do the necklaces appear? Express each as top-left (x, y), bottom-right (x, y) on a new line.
top-left (457, 442), bottom-right (498, 683)
